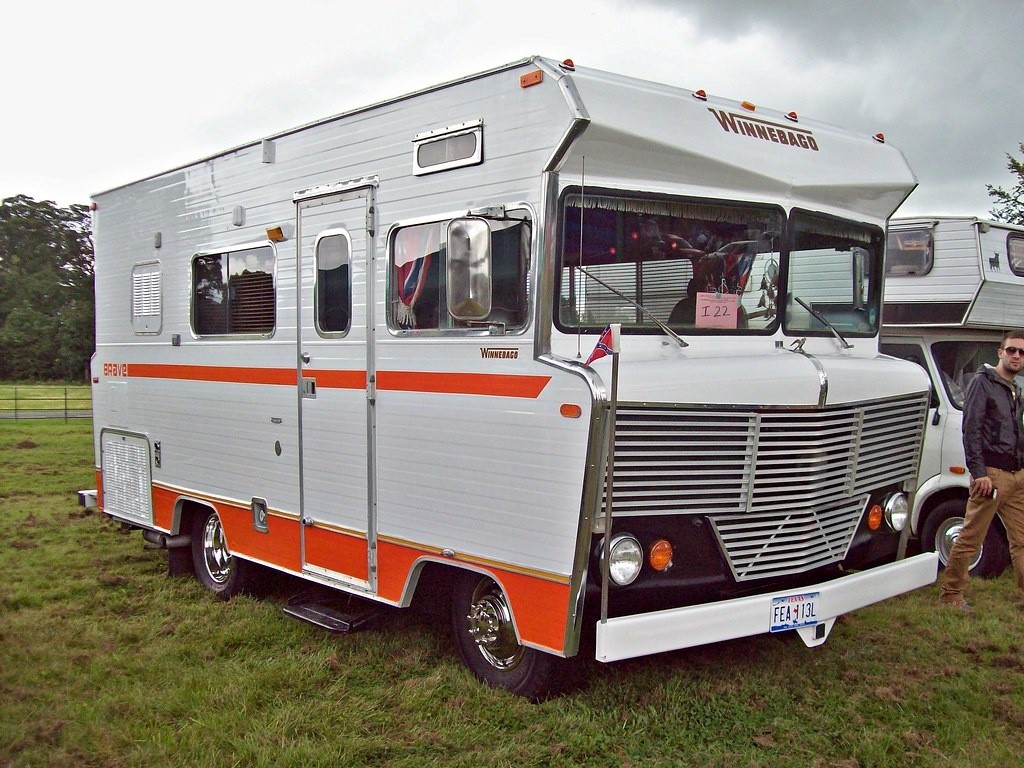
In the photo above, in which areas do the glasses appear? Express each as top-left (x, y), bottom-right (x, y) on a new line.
top-left (1005, 346), bottom-right (1024, 358)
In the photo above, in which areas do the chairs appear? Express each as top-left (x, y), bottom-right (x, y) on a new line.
top-left (668, 274), bottom-right (748, 328)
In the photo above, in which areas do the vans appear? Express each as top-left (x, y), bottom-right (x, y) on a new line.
top-left (524, 214), bottom-right (1024, 584)
top-left (75, 59), bottom-right (941, 704)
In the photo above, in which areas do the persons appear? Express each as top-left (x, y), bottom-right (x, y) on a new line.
top-left (942, 330), bottom-right (1024, 619)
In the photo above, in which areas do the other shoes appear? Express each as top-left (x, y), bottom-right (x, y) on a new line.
top-left (940, 600), bottom-right (970, 611)
